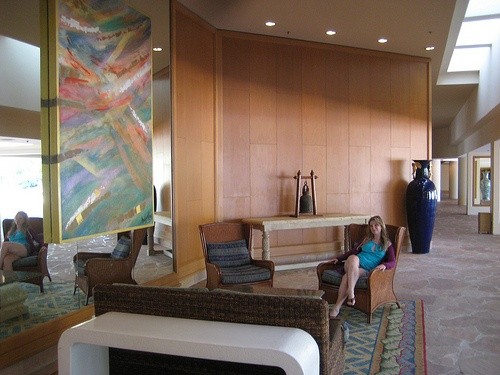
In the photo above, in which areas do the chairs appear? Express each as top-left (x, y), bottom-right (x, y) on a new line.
top-left (198, 222), bottom-right (274, 290)
top-left (73, 228), bottom-right (146, 306)
top-left (1, 217), bottom-right (52, 294)
top-left (316, 223), bottom-right (407, 324)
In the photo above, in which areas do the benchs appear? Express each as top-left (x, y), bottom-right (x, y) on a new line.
top-left (94, 283), bottom-right (346, 375)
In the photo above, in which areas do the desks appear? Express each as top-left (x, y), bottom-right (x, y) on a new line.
top-left (57, 311), bottom-right (320, 375)
top-left (243, 214), bottom-right (371, 271)
top-left (152, 211), bottom-right (173, 259)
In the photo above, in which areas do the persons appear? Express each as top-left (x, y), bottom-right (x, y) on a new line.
top-left (0, 211), bottom-right (42, 271)
top-left (330, 216), bottom-right (396, 317)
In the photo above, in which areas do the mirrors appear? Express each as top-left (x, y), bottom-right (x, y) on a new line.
top-left (473, 156), bottom-right (492, 206)
top-left (0, 0), bottom-right (182, 354)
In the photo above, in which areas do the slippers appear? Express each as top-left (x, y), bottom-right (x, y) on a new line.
top-left (347, 297), bottom-right (356, 306)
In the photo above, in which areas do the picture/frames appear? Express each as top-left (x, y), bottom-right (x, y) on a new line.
top-left (48, 0), bottom-right (154, 243)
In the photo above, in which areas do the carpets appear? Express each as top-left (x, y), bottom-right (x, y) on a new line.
top-left (36, 299), bottom-right (427, 375)
top-left (330, 299), bottom-right (428, 375)
top-left (0, 230), bottom-right (173, 342)
